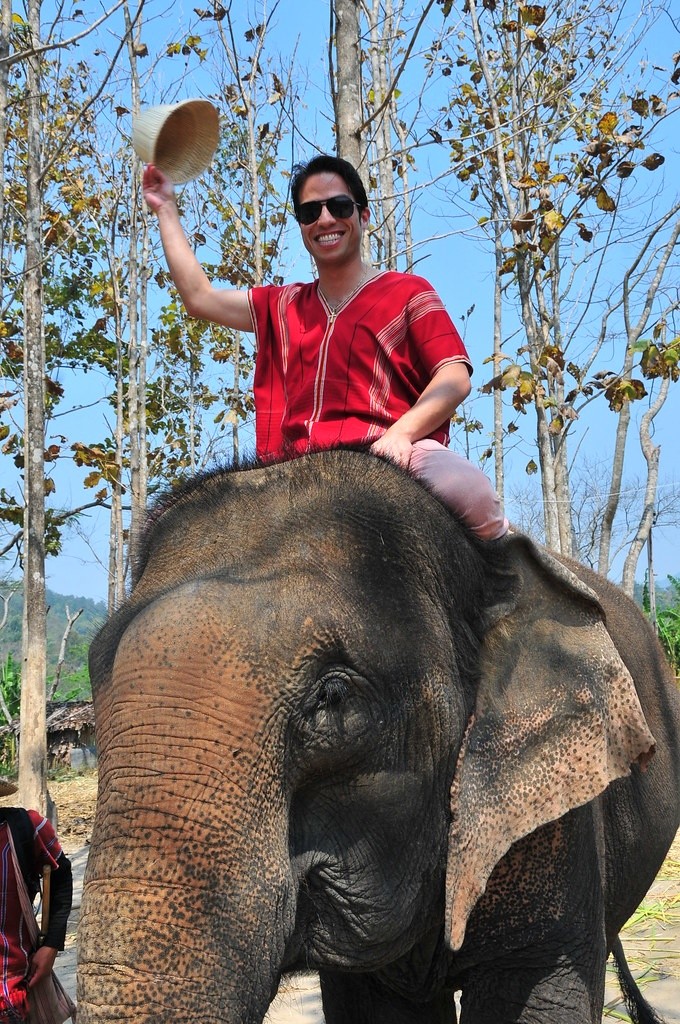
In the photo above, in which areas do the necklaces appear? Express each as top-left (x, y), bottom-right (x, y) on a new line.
top-left (328, 270), bottom-right (367, 323)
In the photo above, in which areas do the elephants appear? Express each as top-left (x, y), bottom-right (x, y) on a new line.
top-left (75, 441), bottom-right (680, 1023)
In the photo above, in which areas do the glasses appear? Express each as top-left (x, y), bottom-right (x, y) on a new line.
top-left (295, 195), bottom-right (361, 225)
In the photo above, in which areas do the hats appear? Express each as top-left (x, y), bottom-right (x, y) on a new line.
top-left (0, 779), bottom-right (19, 797)
top-left (133, 98), bottom-right (219, 183)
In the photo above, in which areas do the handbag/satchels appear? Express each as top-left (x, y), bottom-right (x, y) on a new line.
top-left (21, 938), bottom-right (76, 1024)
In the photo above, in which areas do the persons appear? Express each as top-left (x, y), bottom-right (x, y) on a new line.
top-left (142, 157), bottom-right (514, 544)
top-left (0, 777), bottom-right (73, 1024)
